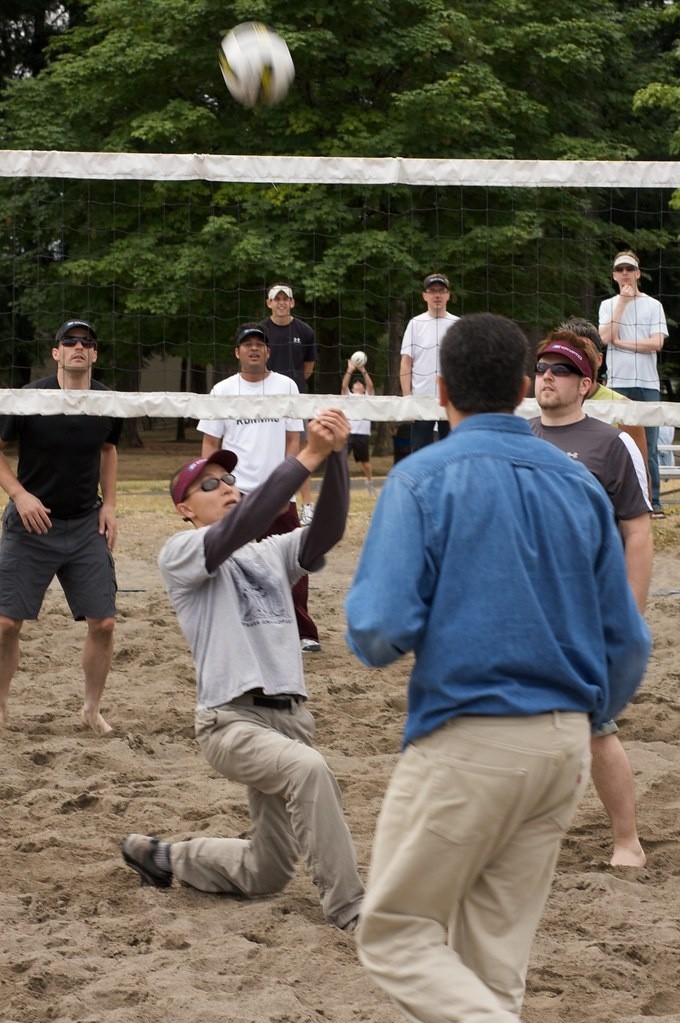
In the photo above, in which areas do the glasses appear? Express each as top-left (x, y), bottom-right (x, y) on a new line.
top-left (181, 474), bottom-right (235, 503)
top-left (534, 363), bottom-right (583, 378)
top-left (61, 336), bottom-right (93, 348)
top-left (616, 265), bottom-right (637, 271)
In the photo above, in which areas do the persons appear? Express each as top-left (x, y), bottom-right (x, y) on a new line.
top-left (340, 309), bottom-right (652, 1023)
top-left (521, 327), bottom-right (650, 618)
top-left (555, 314), bottom-right (647, 478)
top-left (337, 355), bottom-right (378, 490)
top-left (398, 271), bottom-right (461, 451)
top-left (196, 320), bottom-right (326, 654)
top-left (0, 318), bottom-right (116, 738)
top-left (596, 249), bottom-right (669, 522)
top-left (116, 411), bottom-right (370, 931)
top-left (247, 285), bottom-right (318, 527)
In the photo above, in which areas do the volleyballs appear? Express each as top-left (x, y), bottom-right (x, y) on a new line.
top-left (351, 351), bottom-right (368, 368)
top-left (218, 19), bottom-right (296, 110)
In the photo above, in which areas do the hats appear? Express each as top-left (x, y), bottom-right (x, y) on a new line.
top-left (537, 342), bottom-right (592, 380)
top-left (236, 329), bottom-right (265, 346)
top-left (174, 450), bottom-right (237, 505)
top-left (55, 321), bottom-right (98, 342)
top-left (424, 278), bottom-right (447, 289)
top-left (268, 285), bottom-right (292, 301)
top-left (614, 256), bottom-right (638, 269)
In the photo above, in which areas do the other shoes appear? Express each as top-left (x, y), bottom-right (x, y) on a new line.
top-left (301, 639), bottom-right (320, 651)
top-left (121, 833), bottom-right (173, 889)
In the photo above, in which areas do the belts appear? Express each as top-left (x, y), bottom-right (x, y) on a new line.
top-left (253, 696), bottom-right (298, 709)
